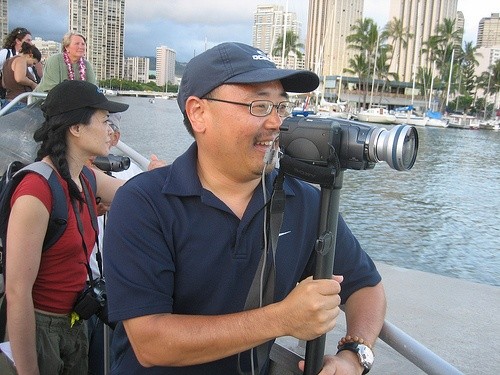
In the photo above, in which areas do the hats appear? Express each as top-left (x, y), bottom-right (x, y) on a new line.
top-left (45, 79), bottom-right (129, 117)
top-left (177, 42), bottom-right (320, 112)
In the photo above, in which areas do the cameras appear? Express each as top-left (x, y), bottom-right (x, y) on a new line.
top-left (89, 152), bottom-right (130, 177)
top-left (276, 110), bottom-right (420, 174)
top-left (71, 275), bottom-right (118, 331)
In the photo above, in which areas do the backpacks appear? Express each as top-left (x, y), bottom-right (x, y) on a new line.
top-left (0, 160), bottom-right (97, 352)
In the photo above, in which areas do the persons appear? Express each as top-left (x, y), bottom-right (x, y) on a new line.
top-left (103, 42), bottom-right (386, 375)
top-left (5, 79), bottom-right (168, 375)
top-left (0, 27), bottom-right (96, 118)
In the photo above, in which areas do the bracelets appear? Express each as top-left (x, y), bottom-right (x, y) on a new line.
top-left (336, 335), bottom-right (373, 349)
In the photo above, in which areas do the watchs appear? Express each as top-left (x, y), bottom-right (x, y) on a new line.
top-left (336, 343), bottom-right (375, 375)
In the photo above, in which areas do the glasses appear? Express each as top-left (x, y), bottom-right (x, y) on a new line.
top-left (13, 28), bottom-right (27, 41)
top-left (199, 96), bottom-right (293, 118)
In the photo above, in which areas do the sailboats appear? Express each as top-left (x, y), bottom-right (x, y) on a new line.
top-left (280, 0), bottom-right (500, 132)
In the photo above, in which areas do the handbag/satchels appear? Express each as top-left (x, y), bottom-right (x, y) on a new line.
top-left (0, 49), bottom-right (10, 99)
